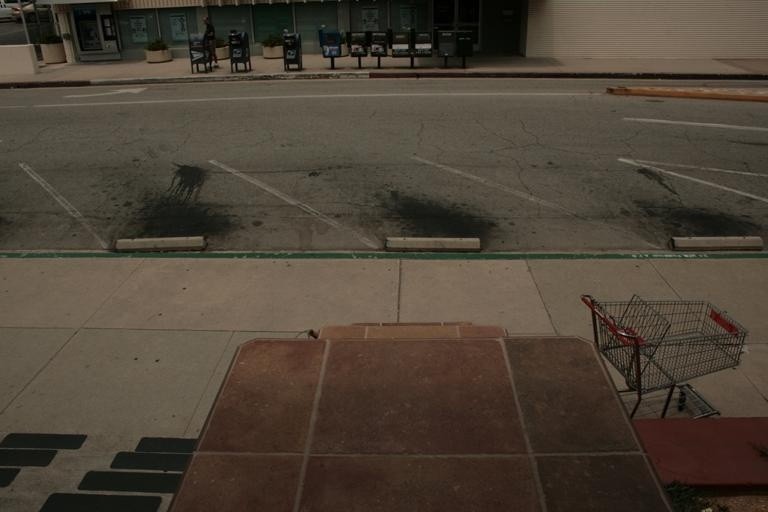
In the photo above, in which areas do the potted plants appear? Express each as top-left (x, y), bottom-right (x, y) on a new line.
top-left (40, 35), bottom-right (67, 64)
top-left (144, 38), bottom-right (172, 62)
top-left (340, 32), bottom-right (349, 56)
top-left (215, 39), bottom-right (230, 60)
top-left (261, 35), bottom-right (284, 58)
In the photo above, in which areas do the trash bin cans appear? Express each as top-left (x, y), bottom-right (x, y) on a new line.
top-left (38, 37), bottom-right (66, 64)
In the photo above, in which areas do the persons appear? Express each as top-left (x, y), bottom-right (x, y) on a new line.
top-left (203, 16), bottom-right (218, 67)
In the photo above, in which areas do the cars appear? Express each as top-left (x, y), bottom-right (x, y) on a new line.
top-left (0, 0), bottom-right (38, 26)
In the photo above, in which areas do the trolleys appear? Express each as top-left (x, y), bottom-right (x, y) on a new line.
top-left (579, 290), bottom-right (751, 419)
